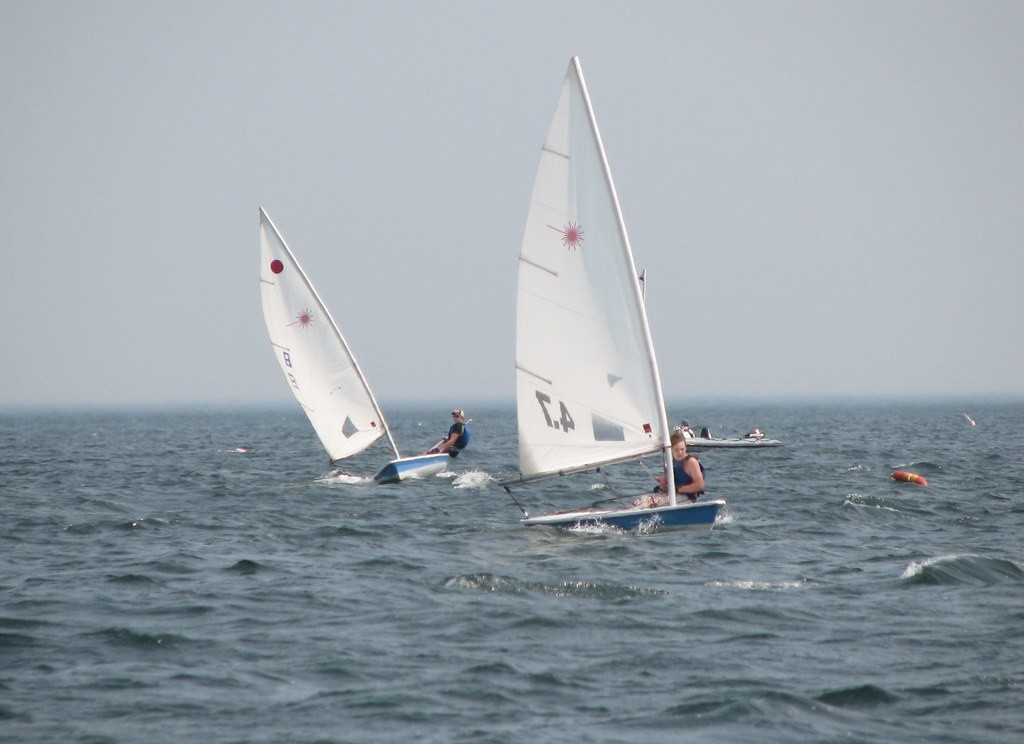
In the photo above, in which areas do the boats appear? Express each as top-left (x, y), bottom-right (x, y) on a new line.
top-left (669, 431), bottom-right (783, 448)
top-left (499, 53), bottom-right (731, 537)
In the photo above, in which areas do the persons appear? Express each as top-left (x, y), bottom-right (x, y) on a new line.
top-left (421, 409), bottom-right (471, 458)
top-left (652, 429), bottom-right (705, 505)
top-left (744, 425), bottom-right (766, 440)
top-left (673, 421), bottom-right (696, 439)
top-left (700, 427), bottom-right (713, 440)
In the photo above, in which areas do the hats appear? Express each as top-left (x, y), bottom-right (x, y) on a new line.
top-left (451, 409), bottom-right (464, 419)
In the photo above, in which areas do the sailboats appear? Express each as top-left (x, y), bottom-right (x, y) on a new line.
top-left (259, 201), bottom-right (451, 485)
